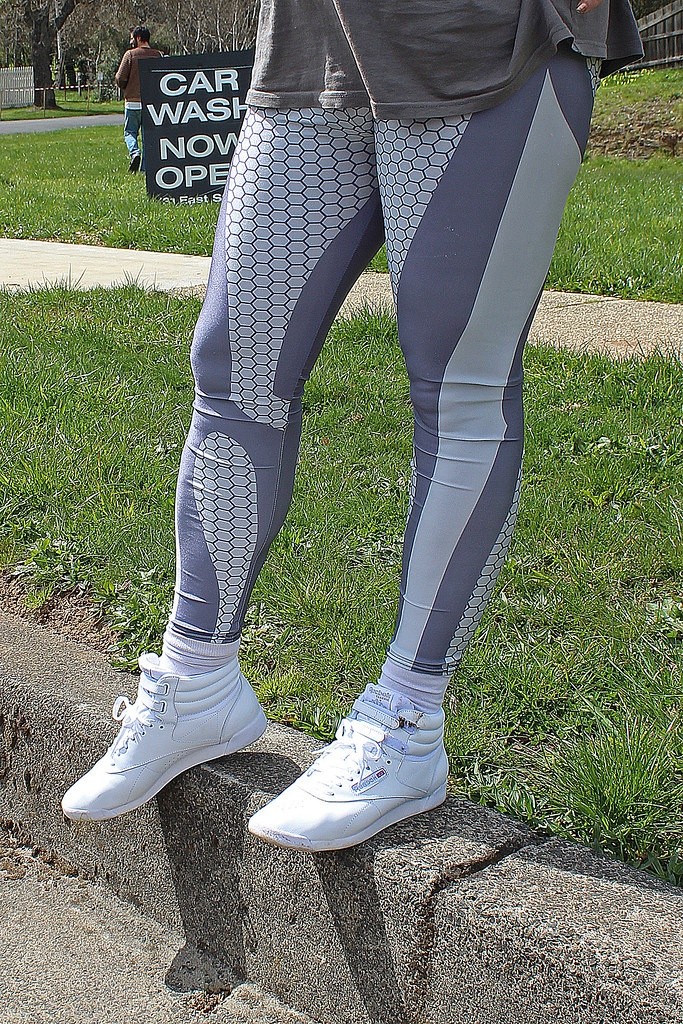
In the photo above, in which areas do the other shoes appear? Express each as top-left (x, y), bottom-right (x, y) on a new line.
top-left (127, 154), bottom-right (141, 171)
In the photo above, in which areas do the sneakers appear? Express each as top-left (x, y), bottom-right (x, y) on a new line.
top-left (248, 683), bottom-right (449, 852)
top-left (62, 652), bottom-right (267, 821)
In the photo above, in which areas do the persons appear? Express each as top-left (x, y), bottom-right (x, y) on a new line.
top-left (115, 25), bottom-right (163, 174)
top-left (61, 0), bottom-right (646, 852)
